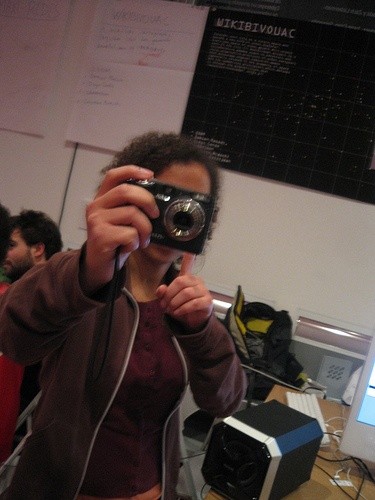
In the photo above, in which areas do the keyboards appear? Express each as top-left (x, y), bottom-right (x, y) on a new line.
top-left (286, 391), bottom-right (330, 447)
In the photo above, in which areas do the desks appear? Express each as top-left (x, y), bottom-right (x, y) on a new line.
top-left (203, 384), bottom-right (375, 500)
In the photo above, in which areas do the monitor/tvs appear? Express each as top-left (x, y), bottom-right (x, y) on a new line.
top-left (339, 331), bottom-right (375, 464)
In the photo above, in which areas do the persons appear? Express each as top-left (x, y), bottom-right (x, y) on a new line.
top-left (2, 208), bottom-right (71, 456)
top-left (0, 129), bottom-right (249, 500)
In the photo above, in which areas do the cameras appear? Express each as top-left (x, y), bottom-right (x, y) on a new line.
top-left (123, 177), bottom-right (215, 255)
